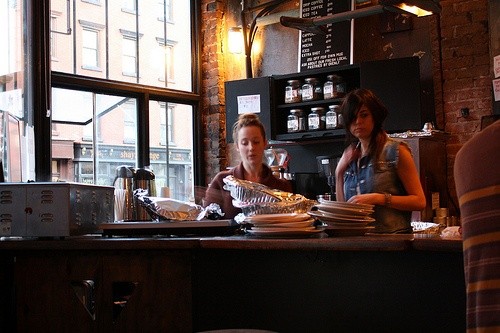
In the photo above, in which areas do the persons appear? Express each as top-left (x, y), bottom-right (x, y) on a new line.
top-left (453, 121), bottom-right (500, 333)
top-left (203, 114), bottom-right (292, 235)
top-left (335, 88), bottom-right (426, 234)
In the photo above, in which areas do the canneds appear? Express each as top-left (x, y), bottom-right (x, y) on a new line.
top-left (326, 105), bottom-right (345, 130)
top-left (323, 75), bottom-right (345, 99)
top-left (287, 109), bottom-right (307, 133)
top-left (308, 107), bottom-right (326, 130)
top-left (284, 79), bottom-right (301, 103)
top-left (302, 78), bottom-right (322, 101)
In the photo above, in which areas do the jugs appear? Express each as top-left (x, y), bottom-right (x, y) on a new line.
top-left (113, 164), bottom-right (156, 222)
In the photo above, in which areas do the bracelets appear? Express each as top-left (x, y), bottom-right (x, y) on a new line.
top-left (385, 194), bottom-right (392, 208)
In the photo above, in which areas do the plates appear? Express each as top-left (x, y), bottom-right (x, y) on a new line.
top-left (244, 200), bottom-right (376, 237)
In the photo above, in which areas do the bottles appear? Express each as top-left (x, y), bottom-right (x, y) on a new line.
top-left (284, 80), bottom-right (301, 104)
top-left (302, 78), bottom-right (322, 102)
top-left (308, 107), bottom-right (326, 131)
top-left (326, 105), bottom-right (345, 130)
top-left (287, 110), bottom-right (306, 134)
top-left (323, 75), bottom-right (345, 99)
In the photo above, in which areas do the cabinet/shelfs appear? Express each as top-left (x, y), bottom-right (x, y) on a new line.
top-left (223, 55), bottom-right (421, 143)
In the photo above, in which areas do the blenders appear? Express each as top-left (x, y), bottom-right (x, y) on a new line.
top-left (316, 152), bottom-right (342, 201)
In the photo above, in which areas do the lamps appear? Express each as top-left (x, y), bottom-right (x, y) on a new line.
top-left (228, 27), bottom-right (244, 54)
top-left (378, 0), bottom-right (438, 17)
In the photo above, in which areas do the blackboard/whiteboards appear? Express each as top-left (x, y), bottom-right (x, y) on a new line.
top-left (297, 0), bottom-right (354, 73)
